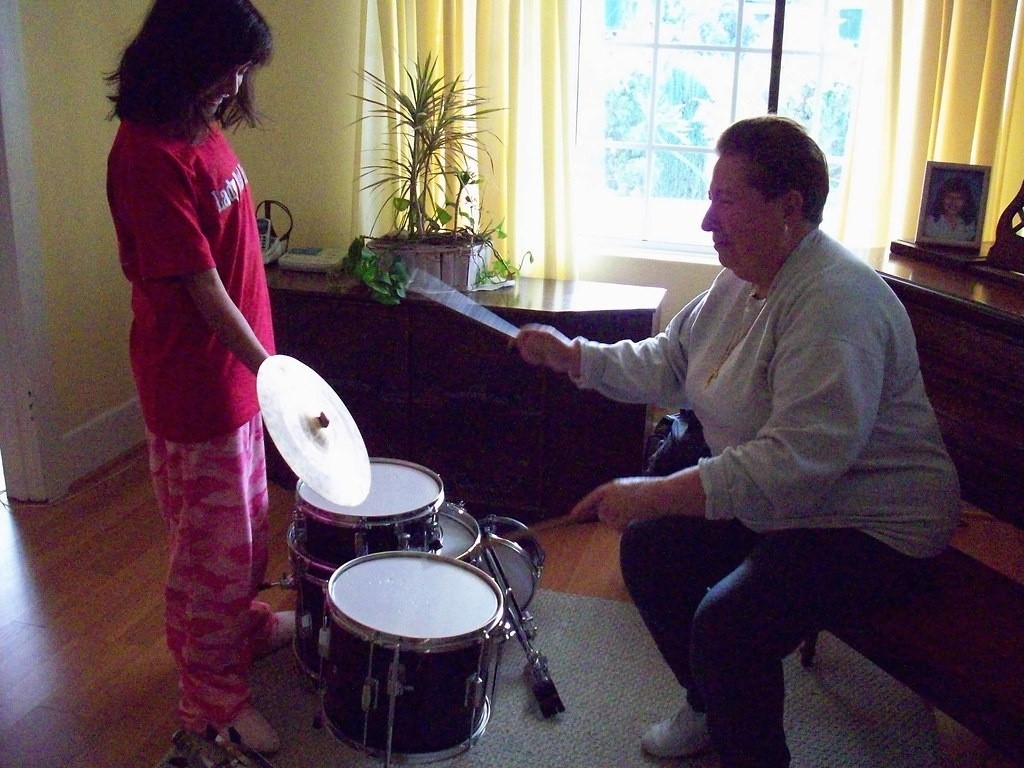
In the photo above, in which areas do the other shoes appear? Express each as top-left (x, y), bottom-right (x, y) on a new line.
top-left (217, 702), bottom-right (282, 754)
top-left (258, 608), bottom-right (296, 659)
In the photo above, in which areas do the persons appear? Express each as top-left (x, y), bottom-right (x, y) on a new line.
top-left (104, 0), bottom-right (298, 756)
top-left (514, 116), bottom-right (959, 767)
top-left (921, 178), bottom-right (978, 242)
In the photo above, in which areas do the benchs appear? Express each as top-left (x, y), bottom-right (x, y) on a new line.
top-left (795, 497), bottom-right (1024, 768)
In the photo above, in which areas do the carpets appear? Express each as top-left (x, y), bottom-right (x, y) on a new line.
top-left (154, 586), bottom-right (937, 768)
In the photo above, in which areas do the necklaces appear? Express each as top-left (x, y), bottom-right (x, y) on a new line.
top-left (707, 281), bottom-right (767, 386)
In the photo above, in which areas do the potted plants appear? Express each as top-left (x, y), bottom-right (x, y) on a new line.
top-left (343, 51), bottom-right (533, 303)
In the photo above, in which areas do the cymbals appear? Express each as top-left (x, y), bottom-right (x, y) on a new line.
top-left (256, 355), bottom-right (373, 508)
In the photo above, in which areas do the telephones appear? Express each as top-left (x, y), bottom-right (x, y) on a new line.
top-left (256, 218), bottom-right (282, 263)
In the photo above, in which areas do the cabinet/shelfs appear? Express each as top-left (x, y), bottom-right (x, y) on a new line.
top-left (268, 269), bottom-right (668, 513)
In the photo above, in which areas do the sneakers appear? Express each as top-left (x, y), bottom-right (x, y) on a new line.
top-left (642, 700), bottom-right (710, 757)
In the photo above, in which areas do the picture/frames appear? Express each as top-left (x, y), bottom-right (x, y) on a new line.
top-left (915, 160), bottom-right (991, 250)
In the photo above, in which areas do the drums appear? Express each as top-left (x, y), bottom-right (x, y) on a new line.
top-left (463, 513), bottom-right (547, 623)
top-left (320, 551), bottom-right (505, 766)
top-left (287, 503), bottom-right (484, 687)
top-left (296, 455), bottom-right (446, 568)
